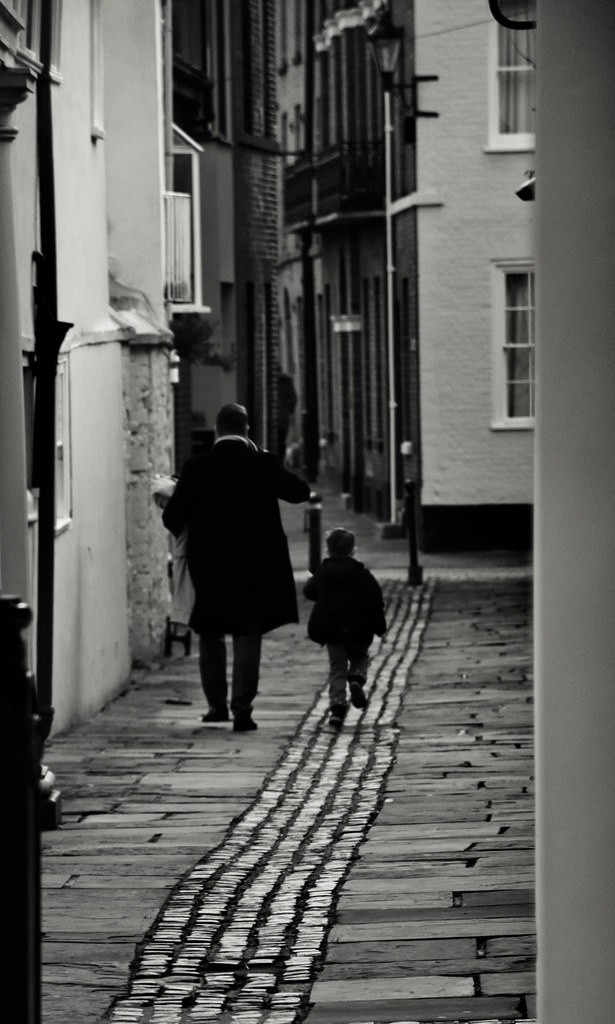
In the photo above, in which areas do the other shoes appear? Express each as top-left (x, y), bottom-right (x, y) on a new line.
top-left (328, 704), bottom-right (346, 726)
top-left (231, 705), bottom-right (258, 731)
top-left (349, 681), bottom-right (366, 708)
top-left (202, 703), bottom-right (229, 722)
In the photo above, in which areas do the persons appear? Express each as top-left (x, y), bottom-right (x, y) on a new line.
top-left (162, 402), bottom-right (312, 733)
top-left (302, 527), bottom-right (387, 725)
top-left (270, 360), bottom-right (298, 464)
